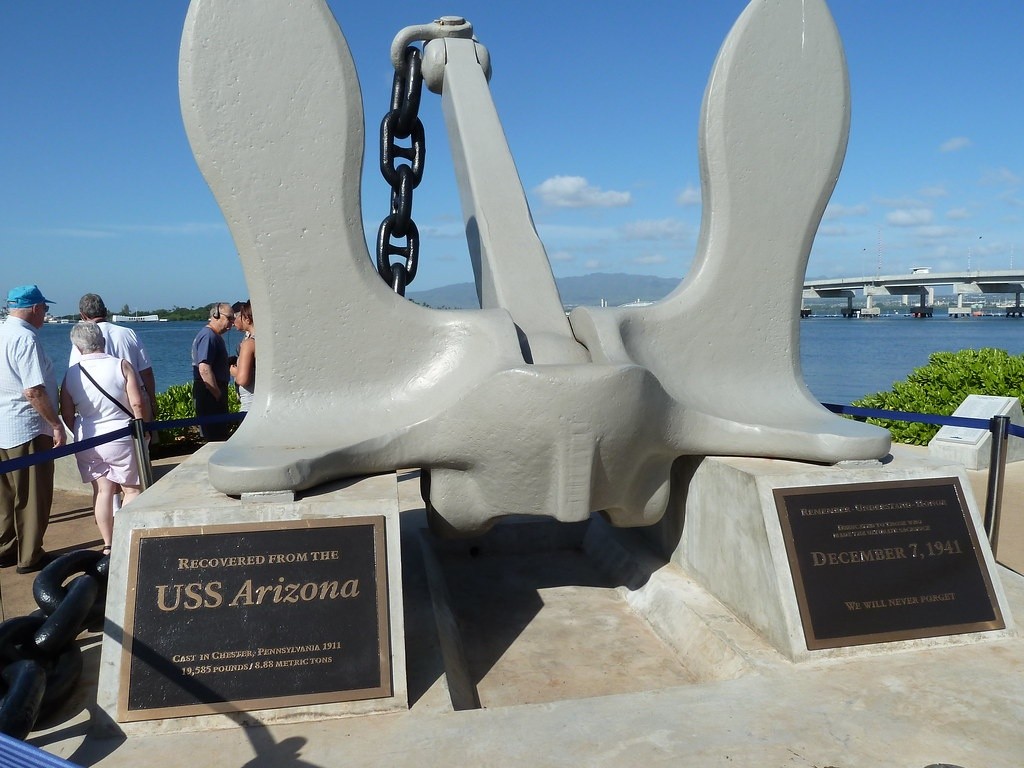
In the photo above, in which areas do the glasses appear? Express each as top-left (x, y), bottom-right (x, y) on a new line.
top-left (219, 313), bottom-right (236, 322)
top-left (40, 306), bottom-right (49, 312)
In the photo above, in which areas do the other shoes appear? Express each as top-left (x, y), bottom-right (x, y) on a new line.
top-left (16, 550), bottom-right (58, 573)
top-left (1, 557), bottom-right (16, 571)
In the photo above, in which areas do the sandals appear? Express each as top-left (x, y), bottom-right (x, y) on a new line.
top-left (101, 546), bottom-right (112, 558)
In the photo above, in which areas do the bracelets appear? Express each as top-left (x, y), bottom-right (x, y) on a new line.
top-left (151, 398), bottom-right (156, 406)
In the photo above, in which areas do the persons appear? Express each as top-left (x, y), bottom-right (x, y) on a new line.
top-left (69, 294), bottom-right (157, 523)
top-left (0, 285), bottom-right (67, 574)
top-left (191, 300), bottom-right (255, 442)
top-left (60, 322), bottom-right (150, 557)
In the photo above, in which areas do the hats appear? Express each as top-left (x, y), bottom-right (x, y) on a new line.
top-left (6, 284), bottom-right (59, 309)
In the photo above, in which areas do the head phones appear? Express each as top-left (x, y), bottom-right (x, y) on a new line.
top-left (213, 303), bottom-right (221, 318)
